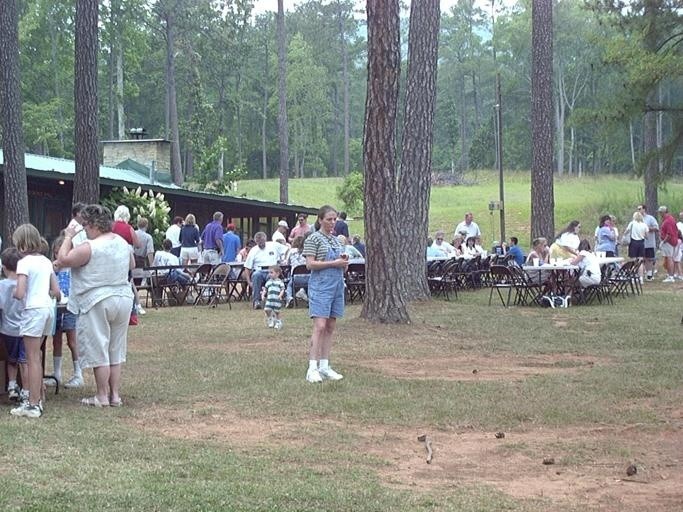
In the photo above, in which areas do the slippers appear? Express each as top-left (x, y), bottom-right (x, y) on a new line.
top-left (81, 395), bottom-right (109, 407)
top-left (111, 397), bottom-right (124, 408)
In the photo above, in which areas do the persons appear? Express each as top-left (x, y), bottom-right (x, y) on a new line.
top-left (455, 212), bottom-right (480, 242)
top-left (334, 212), bottom-right (364, 258)
top-left (154, 211), bottom-right (241, 304)
top-left (56, 204), bottom-right (137, 407)
top-left (1, 201), bottom-right (88, 419)
top-left (525, 219), bottom-right (602, 308)
top-left (108, 205), bottom-right (154, 324)
top-left (302, 204), bottom-right (351, 383)
top-left (624, 204), bottom-right (682, 282)
top-left (237, 213), bottom-right (312, 309)
top-left (594, 214), bottom-right (622, 277)
top-left (261, 265), bottom-right (285, 329)
top-left (427, 230), bottom-right (484, 258)
top-left (501, 237), bottom-right (523, 284)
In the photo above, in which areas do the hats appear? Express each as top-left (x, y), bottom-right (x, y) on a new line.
top-left (655, 206), bottom-right (667, 213)
top-left (278, 220), bottom-right (289, 229)
top-left (227, 223), bottom-right (235, 230)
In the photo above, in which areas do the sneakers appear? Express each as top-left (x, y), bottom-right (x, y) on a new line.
top-left (296, 289), bottom-right (308, 302)
top-left (8, 376), bottom-right (84, 418)
top-left (274, 320), bottom-right (282, 329)
top-left (285, 297), bottom-right (295, 308)
top-left (662, 276), bottom-right (674, 282)
top-left (306, 371), bottom-right (322, 383)
top-left (319, 366), bottom-right (343, 381)
top-left (268, 317), bottom-right (275, 327)
top-left (136, 305), bottom-right (146, 315)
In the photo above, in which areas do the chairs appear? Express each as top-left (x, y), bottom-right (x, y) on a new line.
top-left (132, 258), bottom-right (365, 309)
top-left (425, 247), bottom-right (650, 311)
